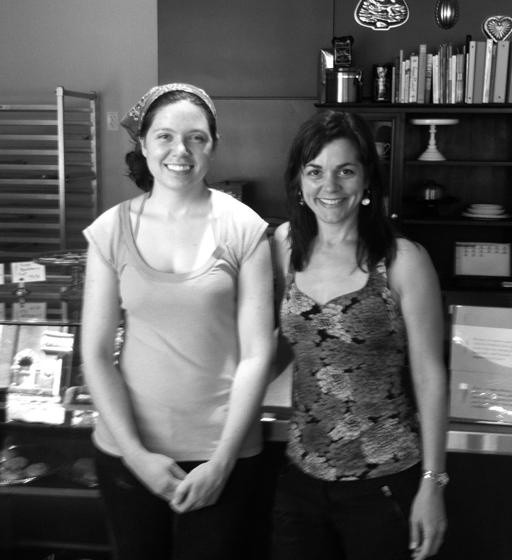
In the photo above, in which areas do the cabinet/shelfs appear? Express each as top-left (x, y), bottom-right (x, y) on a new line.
top-left (0, 87), bottom-right (103, 306)
top-left (316, 96), bottom-right (511, 308)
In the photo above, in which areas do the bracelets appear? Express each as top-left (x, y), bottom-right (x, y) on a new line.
top-left (420, 468), bottom-right (452, 488)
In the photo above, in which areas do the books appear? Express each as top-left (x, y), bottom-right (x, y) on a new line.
top-left (390, 34), bottom-right (511, 104)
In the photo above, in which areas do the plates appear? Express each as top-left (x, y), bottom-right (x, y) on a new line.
top-left (460, 202), bottom-right (512, 220)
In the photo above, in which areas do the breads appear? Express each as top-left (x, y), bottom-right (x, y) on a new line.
top-left (1, 449), bottom-right (48, 482)
top-left (72, 457), bottom-right (98, 488)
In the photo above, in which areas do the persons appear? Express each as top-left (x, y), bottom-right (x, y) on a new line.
top-left (270, 107), bottom-right (451, 560)
top-left (77, 78), bottom-right (278, 559)
